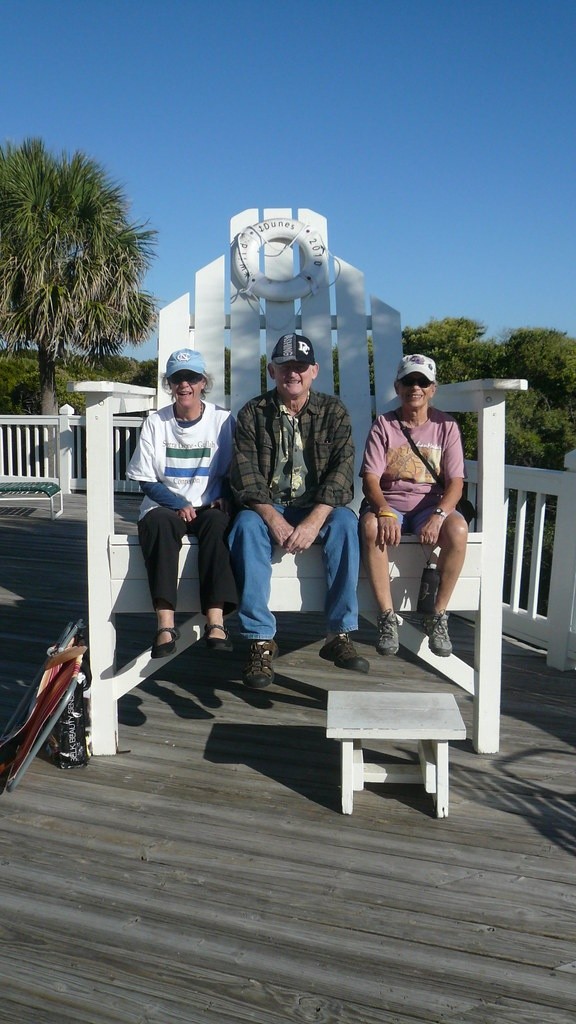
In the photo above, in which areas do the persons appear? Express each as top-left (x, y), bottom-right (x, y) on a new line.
top-left (359, 354), bottom-right (469, 658)
top-left (125, 349), bottom-right (236, 659)
top-left (226, 333), bottom-right (369, 690)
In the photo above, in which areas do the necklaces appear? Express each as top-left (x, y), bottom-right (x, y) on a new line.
top-left (174, 401), bottom-right (202, 416)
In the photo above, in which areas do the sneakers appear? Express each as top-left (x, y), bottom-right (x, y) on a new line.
top-left (244, 639), bottom-right (279, 687)
top-left (376, 608), bottom-right (399, 655)
top-left (422, 610), bottom-right (453, 658)
top-left (319, 631), bottom-right (369, 674)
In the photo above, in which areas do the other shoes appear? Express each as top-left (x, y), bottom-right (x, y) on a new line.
top-left (151, 627), bottom-right (180, 658)
top-left (203, 623), bottom-right (233, 651)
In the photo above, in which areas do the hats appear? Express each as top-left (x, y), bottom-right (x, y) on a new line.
top-left (270, 333), bottom-right (315, 365)
top-left (166, 349), bottom-right (204, 378)
top-left (395, 354), bottom-right (436, 384)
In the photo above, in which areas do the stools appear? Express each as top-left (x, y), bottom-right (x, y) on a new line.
top-left (326, 690), bottom-right (466, 818)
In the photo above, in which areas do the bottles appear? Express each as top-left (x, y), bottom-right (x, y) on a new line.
top-left (416, 568), bottom-right (439, 613)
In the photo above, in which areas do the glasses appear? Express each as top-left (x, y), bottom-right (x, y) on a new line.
top-left (170, 374), bottom-right (204, 384)
top-left (402, 376), bottom-right (433, 389)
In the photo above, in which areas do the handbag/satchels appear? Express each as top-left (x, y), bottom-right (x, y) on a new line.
top-left (417, 568), bottom-right (441, 614)
top-left (454, 500), bottom-right (477, 527)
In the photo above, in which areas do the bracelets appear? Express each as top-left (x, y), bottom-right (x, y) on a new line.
top-left (376, 512), bottom-right (398, 520)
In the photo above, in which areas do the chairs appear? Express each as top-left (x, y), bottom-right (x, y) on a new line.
top-left (72, 207), bottom-right (530, 756)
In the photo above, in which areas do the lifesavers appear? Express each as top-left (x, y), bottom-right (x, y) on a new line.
top-left (232, 217), bottom-right (329, 303)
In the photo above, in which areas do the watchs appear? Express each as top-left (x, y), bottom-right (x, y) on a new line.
top-left (432, 508), bottom-right (448, 519)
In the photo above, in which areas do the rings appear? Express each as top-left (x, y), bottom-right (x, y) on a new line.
top-left (182, 518), bottom-right (187, 522)
